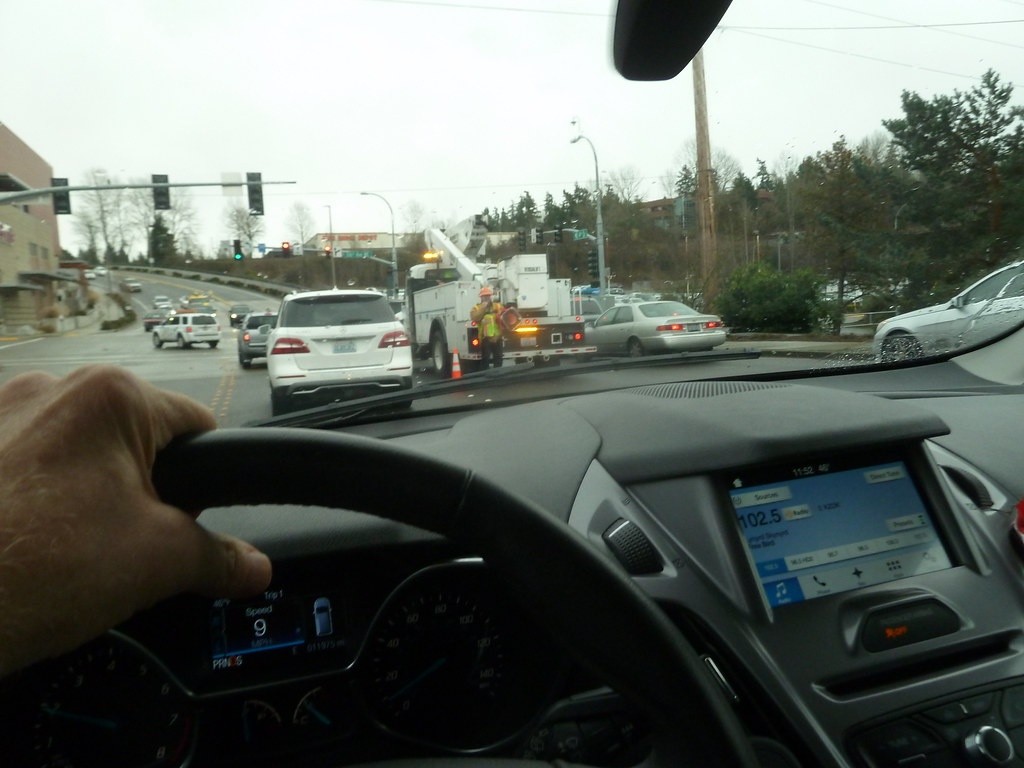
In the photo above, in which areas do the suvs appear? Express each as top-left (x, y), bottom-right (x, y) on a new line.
top-left (267, 286), bottom-right (413, 417)
top-left (152, 313), bottom-right (221, 349)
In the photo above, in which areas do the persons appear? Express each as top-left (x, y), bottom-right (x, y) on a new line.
top-left (0, 364), bottom-right (271, 679)
top-left (470, 288), bottom-right (508, 368)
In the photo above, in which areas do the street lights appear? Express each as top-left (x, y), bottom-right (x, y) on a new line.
top-left (361, 192), bottom-right (399, 286)
top-left (570, 134), bottom-right (606, 298)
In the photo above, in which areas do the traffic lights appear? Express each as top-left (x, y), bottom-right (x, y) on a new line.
top-left (536, 227), bottom-right (543, 245)
top-left (282, 242), bottom-right (290, 258)
top-left (518, 230), bottom-right (526, 252)
top-left (233, 239), bottom-right (242, 260)
top-left (554, 224), bottom-right (562, 242)
top-left (588, 250), bottom-right (599, 278)
top-left (325, 246), bottom-right (331, 260)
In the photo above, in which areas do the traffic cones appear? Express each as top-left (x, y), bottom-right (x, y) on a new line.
top-left (449, 347), bottom-right (463, 380)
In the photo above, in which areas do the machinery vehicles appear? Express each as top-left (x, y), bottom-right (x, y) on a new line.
top-left (400, 215), bottom-right (597, 377)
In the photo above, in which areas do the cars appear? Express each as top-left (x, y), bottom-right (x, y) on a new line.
top-left (821, 280), bottom-right (855, 311)
top-left (119, 278), bottom-right (141, 292)
top-left (94, 266), bottom-right (107, 276)
top-left (575, 301), bottom-right (727, 362)
top-left (874, 259), bottom-right (1024, 364)
top-left (237, 312), bottom-right (278, 368)
top-left (571, 283), bottom-right (703, 327)
top-left (84, 270), bottom-right (96, 279)
top-left (229, 305), bottom-right (252, 327)
top-left (144, 292), bottom-right (217, 332)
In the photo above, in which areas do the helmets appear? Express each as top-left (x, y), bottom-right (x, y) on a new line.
top-left (479, 286), bottom-right (494, 297)
